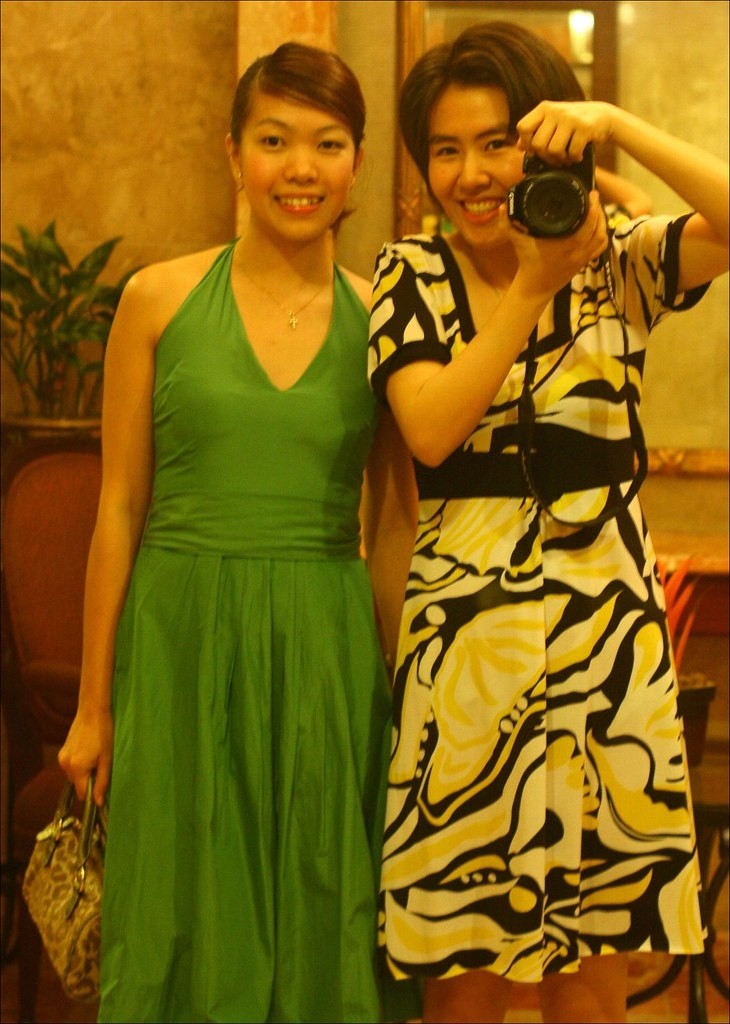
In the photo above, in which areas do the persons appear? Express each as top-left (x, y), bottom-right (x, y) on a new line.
top-left (59, 41), bottom-right (419, 1024)
top-left (365, 21), bottom-right (730, 1024)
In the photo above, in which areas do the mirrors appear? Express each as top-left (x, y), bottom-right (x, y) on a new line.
top-left (394, 1), bottom-right (620, 243)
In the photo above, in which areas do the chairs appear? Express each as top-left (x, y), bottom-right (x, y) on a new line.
top-left (1, 448), bottom-right (104, 1023)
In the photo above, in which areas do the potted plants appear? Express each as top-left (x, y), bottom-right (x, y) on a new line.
top-left (654, 551), bottom-right (718, 771)
top-left (1, 219), bottom-right (150, 482)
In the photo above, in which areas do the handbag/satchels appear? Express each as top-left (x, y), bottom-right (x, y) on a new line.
top-left (19, 775), bottom-right (111, 1003)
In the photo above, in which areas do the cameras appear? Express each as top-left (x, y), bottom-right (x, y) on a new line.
top-left (505, 136), bottom-right (596, 240)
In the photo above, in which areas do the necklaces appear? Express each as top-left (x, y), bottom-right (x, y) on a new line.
top-left (478, 266), bottom-right (504, 300)
top-left (237, 245), bottom-right (333, 330)
